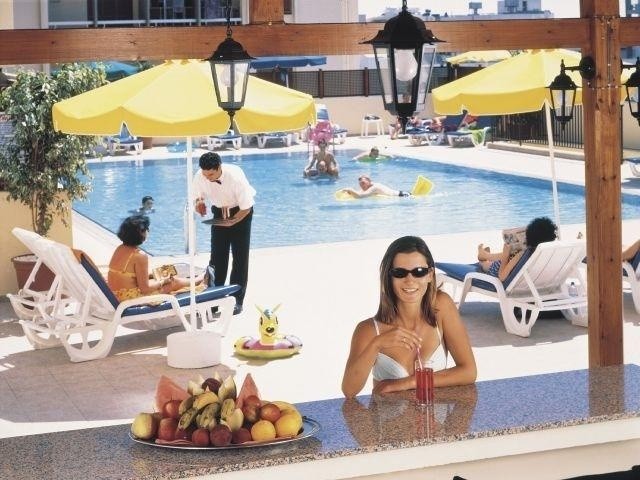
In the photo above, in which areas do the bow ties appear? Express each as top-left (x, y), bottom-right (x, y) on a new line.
top-left (211, 180), bottom-right (222, 184)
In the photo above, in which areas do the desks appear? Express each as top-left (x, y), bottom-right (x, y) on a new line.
top-left (361, 116), bottom-right (384, 136)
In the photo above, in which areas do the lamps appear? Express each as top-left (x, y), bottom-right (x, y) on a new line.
top-left (621, 57), bottom-right (640, 126)
top-left (201, 0), bottom-right (256, 130)
top-left (358, 0), bottom-right (446, 135)
top-left (545, 56), bottom-right (596, 130)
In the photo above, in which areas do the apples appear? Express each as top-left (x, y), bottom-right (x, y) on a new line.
top-left (261, 404), bottom-right (281, 421)
top-left (246, 395), bottom-right (262, 409)
top-left (243, 406), bottom-right (259, 422)
top-left (163, 401), bottom-right (181, 419)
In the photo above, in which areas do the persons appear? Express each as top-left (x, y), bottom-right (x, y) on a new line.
top-left (424, 117), bottom-right (441, 132)
top-left (108, 216), bottom-right (206, 306)
top-left (478, 217), bottom-right (559, 282)
top-left (406, 115), bottom-right (422, 128)
top-left (456, 114), bottom-right (478, 131)
top-left (129, 196), bottom-right (155, 216)
top-left (342, 236), bottom-right (477, 398)
top-left (341, 174), bottom-right (412, 198)
top-left (353, 147), bottom-right (392, 160)
top-left (193, 152), bottom-right (254, 315)
top-left (316, 160), bottom-right (330, 176)
top-left (390, 117), bottom-right (403, 139)
top-left (410, 116), bottom-right (423, 128)
top-left (304, 139), bottom-right (339, 178)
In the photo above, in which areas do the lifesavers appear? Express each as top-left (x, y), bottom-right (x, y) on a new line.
top-left (236, 303), bottom-right (301, 357)
top-left (359, 155), bottom-right (391, 162)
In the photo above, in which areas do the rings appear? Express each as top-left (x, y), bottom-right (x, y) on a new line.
top-left (403, 337), bottom-right (406, 343)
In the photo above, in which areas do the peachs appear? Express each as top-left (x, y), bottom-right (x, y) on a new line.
top-left (158, 419), bottom-right (178, 441)
top-left (175, 427), bottom-right (191, 443)
top-left (281, 408), bottom-right (305, 427)
top-left (210, 425), bottom-right (232, 447)
top-left (192, 429), bottom-right (210, 446)
top-left (232, 428), bottom-right (252, 444)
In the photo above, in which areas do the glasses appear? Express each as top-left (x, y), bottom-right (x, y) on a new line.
top-left (388, 267), bottom-right (432, 278)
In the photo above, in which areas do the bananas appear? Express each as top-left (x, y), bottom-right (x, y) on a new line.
top-left (199, 402), bottom-right (220, 427)
top-left (221, 398), bottom-right (234, 420)
top-left (193, 392), bottom-right (220, 409)
top-left (178, 407), bottom-right (197, 431)
top-left (178, 394), bottom-right (194, 415)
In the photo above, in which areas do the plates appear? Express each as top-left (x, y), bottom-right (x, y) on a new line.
top-left (201, 218), bottom-right (238, 228)
top-left (126, 407), bottom-right (322, 452)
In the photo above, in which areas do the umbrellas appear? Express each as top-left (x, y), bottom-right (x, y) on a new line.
top-left (431, 48), bottom-right (636, 238)
top-left (447, 50), bottom-right (512, 64)
top-left (52, 59), bottom-right (314, 331)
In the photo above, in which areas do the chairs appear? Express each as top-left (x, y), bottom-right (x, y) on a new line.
top-left (194, 133), bottom-right (243, 151)
top-left (405, 109), bottom-right (467, 145)
top-left (622, 157), bottom-right (640, 177)
top-left (434, 239), bottom-right (588, 338)
top-left (389, 118), bottom-right (432, 140)
top-left (103, 135), bottom-right (144, 155)
top-left (243, 133), bottom-right (292, 149)
top-left (6, 227), bottom-right (241, 362)
top-left (315, 104), bottom-right (348, 145)
top-left (622, 249), bottom-right (640, 314)
top-left (446, 116), bottom-right (499, 148)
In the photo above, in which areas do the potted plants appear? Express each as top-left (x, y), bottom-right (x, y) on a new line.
top-left (0, 62), bottom-right (110, 309)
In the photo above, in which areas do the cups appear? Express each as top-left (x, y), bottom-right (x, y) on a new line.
top-left (222, 207), bottom-right (230, 219)
top-left (198, 200), bottom-right (207, 218)
top-left (414, 368), bottom-right (435, 405)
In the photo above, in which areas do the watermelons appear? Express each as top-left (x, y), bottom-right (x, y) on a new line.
top-left (236, 373), bottom-right (259, 408)
top-left (155, 375), bottom-right (191, 412)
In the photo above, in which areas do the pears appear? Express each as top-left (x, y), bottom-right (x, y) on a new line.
top-left (251, 421), bottom-right (276, 441)
top-left (131, 413), bottom-right (155, 440)
top-left (276, 416), bottom-right (301, 438)
